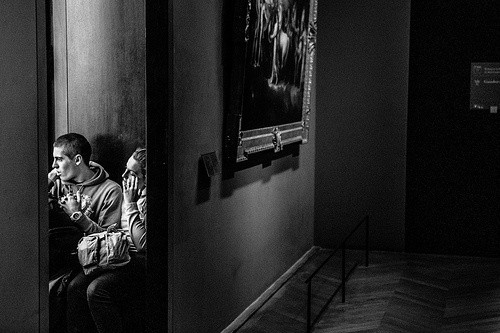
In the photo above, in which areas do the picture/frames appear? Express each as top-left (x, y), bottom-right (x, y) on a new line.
top-left (225, 1), bottom-right (319, 164)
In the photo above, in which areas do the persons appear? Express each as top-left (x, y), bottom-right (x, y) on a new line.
top-left (66, 147), bottom-right (147, 333)
top-left (48, 133), bottom-right (123, 333)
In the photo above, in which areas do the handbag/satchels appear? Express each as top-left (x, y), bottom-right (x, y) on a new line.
top-left (76, 226), bottom-right (132, 276)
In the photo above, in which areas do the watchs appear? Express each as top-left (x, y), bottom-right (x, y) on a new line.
top-left (69, 210), bottom-right (83, 222)
